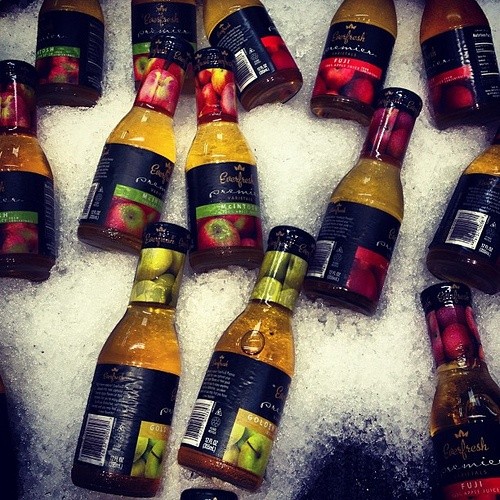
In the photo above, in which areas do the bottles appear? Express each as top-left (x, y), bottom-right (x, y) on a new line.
top-left (35, 0), bottom-right (104, 108)
top-left (175, 226), bottom-right (317, 492)
top-left (427, 137), bottom-right (500, 297)
top-left (300, 86), bottom-right (423, 317)
top-left (184, 47), bottom-right (264, 273)
top-left (78, 35), bottom-right (192, 261)
top-left (131, 0), bottom-right (197, 97)
top-left (203, 0), bottom-right (304, 112)
top-left (420, 0), bottom-right (500, 130)
top-left (70, 221), bottom-right (188, 499)
top-left (179, 488), bottom-right (239, 500)
top-left (0, 60), bottom-right (56, 284)
top-left (420, 282), bottom-right (500, 500)
top-left (309, 0), bottom-right (397, 130)
